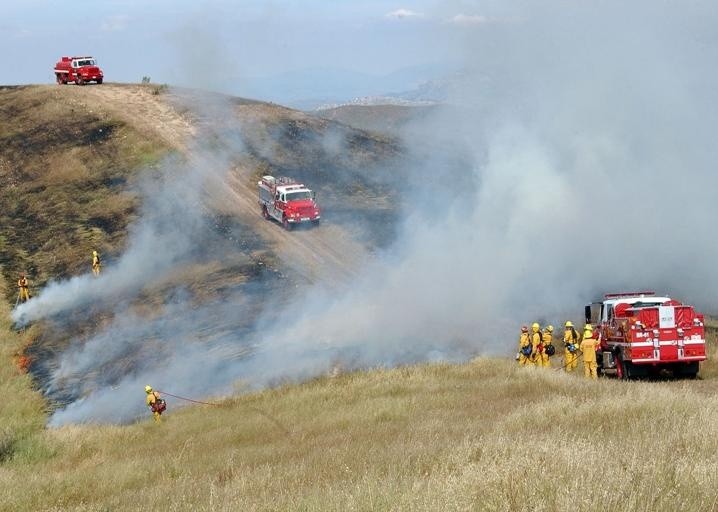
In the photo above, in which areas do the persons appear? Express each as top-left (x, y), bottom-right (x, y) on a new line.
top-left (593, 325), bottom-right (609, 377)
top-left (583, 323), bottom-right (592, 334)
top-left (531, 322), bottom-right (542, 368)
top-left (16, 272), bottom-right (30, 303)
top-left (144, 384), bottom-right (164, 422)
top-left (562, 320), bottom-right (581, 372)
top-left (515, 325), bottom-right (533, 367)
top-left (542, 325), bottom-right (554, 367)
top-left (91, 251), bottom-right (100, 277)
top-left (579, 331), bottom-right (603, 380)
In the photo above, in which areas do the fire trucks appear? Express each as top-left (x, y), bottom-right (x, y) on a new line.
top-left (256, 173), bottom-right (321, 229)
top-left (54, 54), bottom-right (107, 85)
top-left (578, 289), bottom-right (708, 379)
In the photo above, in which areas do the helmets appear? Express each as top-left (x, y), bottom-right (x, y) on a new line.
top-left (584, 324), bottom-right (593, 331)
top-left (144, 385), bottom-right (152, 393)
top-left (583, 331), bottom-right (593, 338)
top-left (531, 322), bottom-right (540, 328)
top-left (521, 326), bottom-right (528, 332)
top-left (565, 321), bottom-right (575, 327)
top-left (546, 326), bottom-right (554, 332)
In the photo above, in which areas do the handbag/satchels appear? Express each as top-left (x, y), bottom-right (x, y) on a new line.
top-left (538, 343), bottom-right (543, 352)
top-left (152, 399), bottom-right (166, 412)
top-left (546, 345), bottom-right (555, 356)
top-left (521, 344), bottom-right (531, 355)
